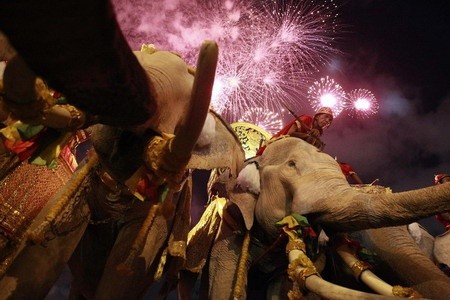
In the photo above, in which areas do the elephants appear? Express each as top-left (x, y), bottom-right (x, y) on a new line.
top-left (179, 134), bottom-right (447, 297)
top-left (0, 3), bottom-right (252, 296)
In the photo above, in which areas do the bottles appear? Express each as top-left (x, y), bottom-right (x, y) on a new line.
top-left (434, 174), bottom-right (449, 184)
top-left (316, 107), bottom-right (333, 116)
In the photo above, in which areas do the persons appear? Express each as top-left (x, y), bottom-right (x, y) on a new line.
top-left (256, 107), bottom-right (333, 155)
top-left (434, 173), bottom-right (450, 230)
top-left (339, 162), bottom-right (363, 184)
